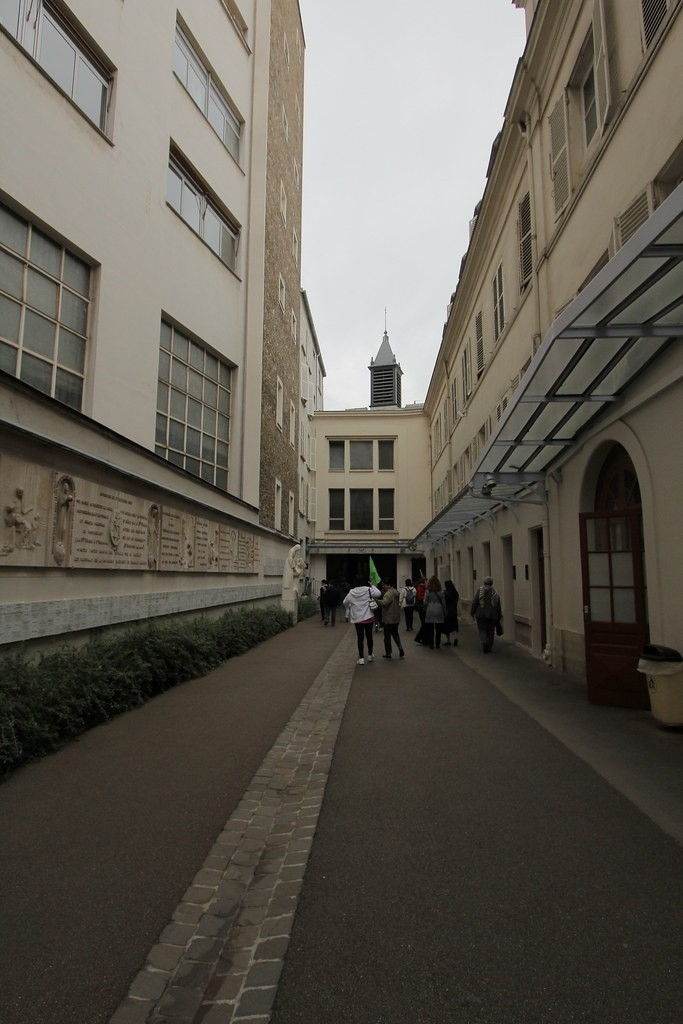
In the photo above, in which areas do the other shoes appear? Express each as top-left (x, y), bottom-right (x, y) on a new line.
top-left (399, 646), bottom-right (404, 657)
top-left (406, 628), bottom-right (409, 631)
top-left (414, 637), bottom-right (458, 650)
top-left (322, 618), bottom-right (349, 626)
top-left (482, 642), bottom-right (493, 654)
top-left (375, 625), bottom-right (378, 632)
top-left (382, 654), bottom-right (391, 659)
top-left (379, 628), bottom-right (384, 632)
top-left (411, 628), bottom-right (413, 631)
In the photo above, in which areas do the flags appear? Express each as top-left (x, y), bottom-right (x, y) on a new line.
top-left (369, 556), bottom-right (381, 587)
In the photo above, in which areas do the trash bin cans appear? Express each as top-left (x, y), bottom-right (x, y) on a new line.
top-left (459, 598), bottom-right (473, 624)
top-left (635, 643), bottom-right (683, 728)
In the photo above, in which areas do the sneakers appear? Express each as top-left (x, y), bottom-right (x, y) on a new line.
top-left (368, 654), bottom-right (375, 662)
top-left (356, 658), bottom-right (365, 665)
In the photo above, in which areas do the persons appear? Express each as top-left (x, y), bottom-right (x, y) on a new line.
top-left (5, 488), bottom-right (42, 548)
top-left (317, 575), bottom-right (458, 664)
top-left (57, 484), bottom-right (74, 543)
top-left (282, 544), bottom-right (302, 589)
top-left (469, 576), bottom-right (503, 654)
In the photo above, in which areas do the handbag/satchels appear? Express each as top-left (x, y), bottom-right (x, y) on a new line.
top-left (496, 620), bottom-right (504, 636)
top-left (368, 587), bottom-right (378, 610)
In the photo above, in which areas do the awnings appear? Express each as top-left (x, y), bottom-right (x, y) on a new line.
top-left (403, 183), bottom-right (683, 558)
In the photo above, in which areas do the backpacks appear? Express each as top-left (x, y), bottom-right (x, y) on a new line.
top-left (403, 587), bottom-right (415, 604)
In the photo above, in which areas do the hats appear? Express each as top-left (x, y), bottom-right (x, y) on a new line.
top-left (483, 576), bottom-right (493, 584)
